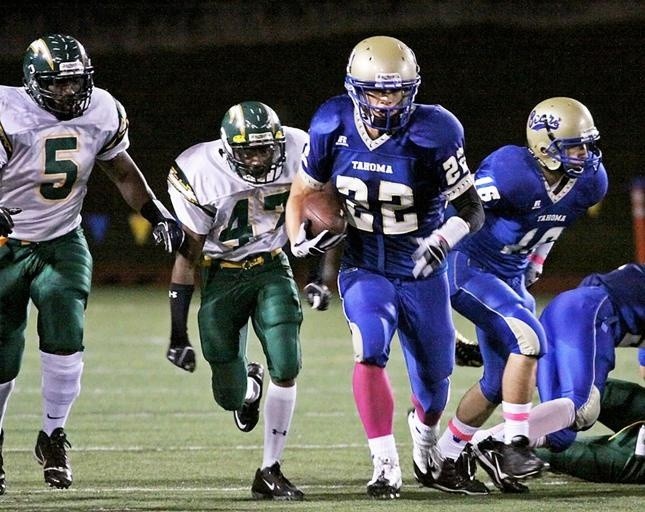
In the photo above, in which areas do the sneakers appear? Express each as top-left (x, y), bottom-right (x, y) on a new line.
top-left (455, 332), bottom-right (483, 367)
top-left (233, 361), bottom-right (265, 432)
top-left (366, 442), bottom-right (402, 499)
top-left (407, 408), bottom-right (545, 496)
top-left (251, 461), bottom-right (304, 500)
top-left (33, 427), bottom-right (72, 488)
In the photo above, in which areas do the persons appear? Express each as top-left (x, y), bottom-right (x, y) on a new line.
top-left (431, 94), bottom-right (609, 497)
top-left (456, 262), bottom-right (644, 492)
top-left (166, 100), bottom-right (332, 506)
top-left (285, 35), bottom-right (488, 500)
top-left (533, 380), bottom-right (644, 486)
top-left (1, 35), bottom-right (190, 498)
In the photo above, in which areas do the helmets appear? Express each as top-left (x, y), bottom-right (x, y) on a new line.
top-left (22, 33), bottom-right (95, 119)
top-left (525, 95), bottom-right (603, 181)
top-left (219, 101), bottom-right (287, 184)
top-left (345, 36), bottom-right (423, 135)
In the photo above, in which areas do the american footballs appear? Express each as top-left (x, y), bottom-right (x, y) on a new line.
top-left (301, 191), bottom-right (346, 239)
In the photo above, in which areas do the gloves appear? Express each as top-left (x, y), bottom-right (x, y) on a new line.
top-left (409, 231), bottom-right (452, 278)
top-left (151, 219), bottom-right (187, 256)
top-left (302, 280), bottom-right (333, 310)
top-left (291, 219), bottom-right (347, 258)
top-left (0, 206), bottom-right (22, 237)
top-left (167, 330), bottom-right (196, 373)
